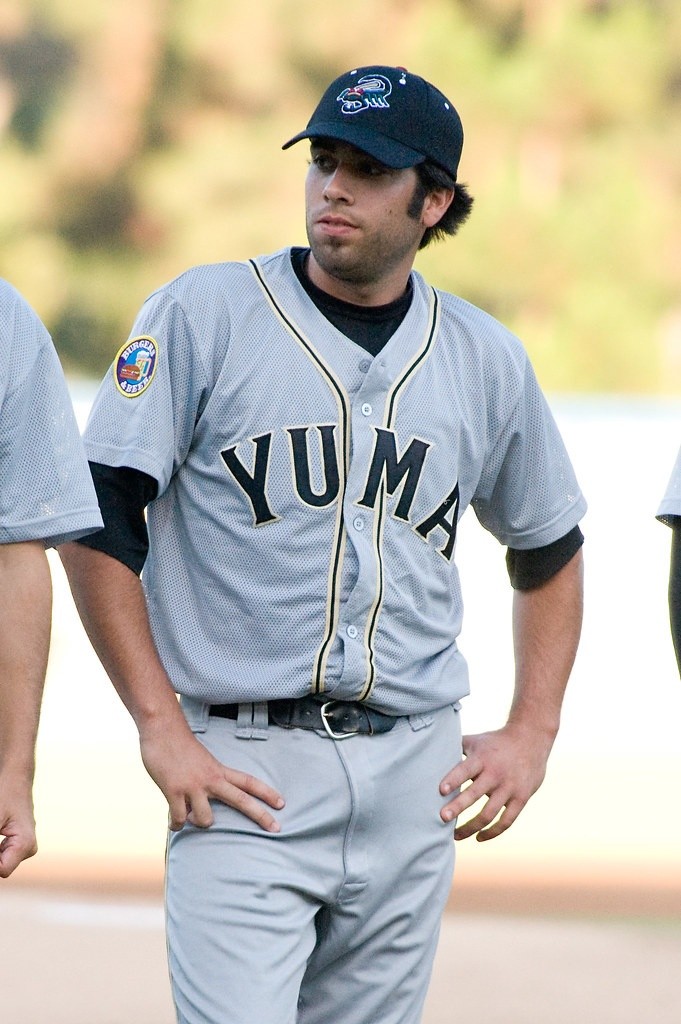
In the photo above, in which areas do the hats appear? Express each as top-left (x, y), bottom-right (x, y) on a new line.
top-left (282, 66), bottom-right (464, 182)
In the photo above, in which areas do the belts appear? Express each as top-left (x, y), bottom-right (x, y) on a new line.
top-left (208, 693), bottom-right (397, 740)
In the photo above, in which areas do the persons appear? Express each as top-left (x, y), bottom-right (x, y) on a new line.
top-left (60, 66), bottom-right (589, 1024)
top-left (654, 450), bottom-right (681, 680)
top-left (0, 279), bottom-right (104, 878)
